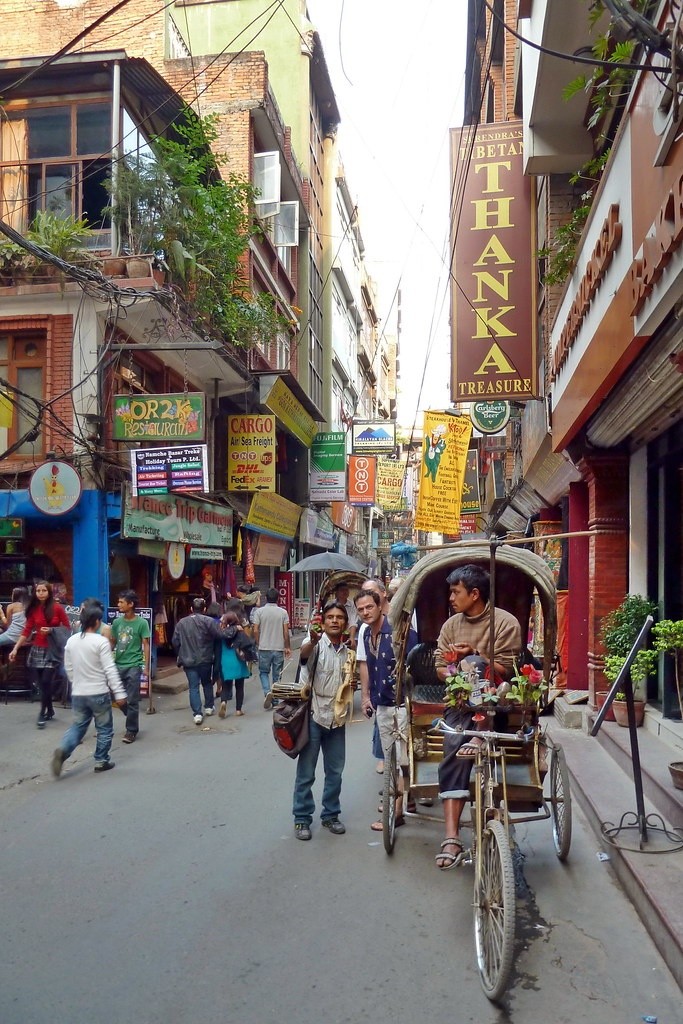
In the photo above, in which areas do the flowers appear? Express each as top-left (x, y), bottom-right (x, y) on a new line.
top-left (440, 652), bottom-right (548, 731)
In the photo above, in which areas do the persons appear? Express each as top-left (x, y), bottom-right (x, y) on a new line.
top-left (171, 584), bottom-right (294, 725)
top-left (292, 602), bottom-right (359, 841)
top-left (48, 605), bottom-right (128, 779)
top-left (435, 564), bottom-right (522, 873)
top-left (77, 590), bottom-right (151, 744)
top-left (9, 580), bottom-right (71, 730)
top-left (332, 574), bottom-right (433, 832)
top-left (322, 580), bottom-right (359, 648)
top-left (0, 586), bottom-right (32, 645)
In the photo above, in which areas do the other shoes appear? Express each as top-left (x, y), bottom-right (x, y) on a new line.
top-left (36, 714), bottom-right (46, 729)
top-left (45, 710), bottom-right (55, 719)
top-left (236, 711), bottom-right (245, 716)
top-left (218, 701), bottom-right (226, 717)
top-left (194, 714), bottom-right (204, 723)
top-left (205, 706), bottom-right (215, 716)
top-left (263, 692), bottom-right (272, 709)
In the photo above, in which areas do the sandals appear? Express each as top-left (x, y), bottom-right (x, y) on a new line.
top-left (435, 838), bottom-right (469, 871)
top-left (371, 813), bottom-right (405, 831)
top-left (377, 800), bottom-right (418, 813)
top-left (456, 736), bottom-right (482, 760)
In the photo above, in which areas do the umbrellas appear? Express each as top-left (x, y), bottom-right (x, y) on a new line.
top-left (286, 551), bottom-right (366, 580)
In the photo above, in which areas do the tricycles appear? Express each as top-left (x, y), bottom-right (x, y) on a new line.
top-left (376, 526), bottom-right (596, 1002)
top-left (305, 570), bottom-right (372, 692)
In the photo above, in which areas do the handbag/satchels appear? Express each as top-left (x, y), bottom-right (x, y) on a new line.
top-left (272, 701), bottom-right (310, 759)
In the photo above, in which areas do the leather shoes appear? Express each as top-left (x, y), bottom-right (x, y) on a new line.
top-left (51, 749), bottom-right (65, 775)
top-left (94, 762), bottom-right (115, 772)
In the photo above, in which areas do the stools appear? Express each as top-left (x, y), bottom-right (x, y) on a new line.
top-left (0, 644), bottom-right (33, 705)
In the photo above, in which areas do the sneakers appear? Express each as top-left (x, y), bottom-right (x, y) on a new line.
top-left (294, 823), bottom-right (312, 839)
top-left (321, 816), bottom-right (346, 833)
top-left (122, 730), bottom-right (138, 743)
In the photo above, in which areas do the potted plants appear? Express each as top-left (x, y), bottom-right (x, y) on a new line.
top-left (650, 619), bottom-right (683, 790)
top-left (0, 101), bottom-right (302, 352)
top-left (587, 594), bottom-right (663, 728)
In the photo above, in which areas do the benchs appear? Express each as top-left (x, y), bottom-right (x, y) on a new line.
top-left (406, 642), bottom-right (546, 722)
